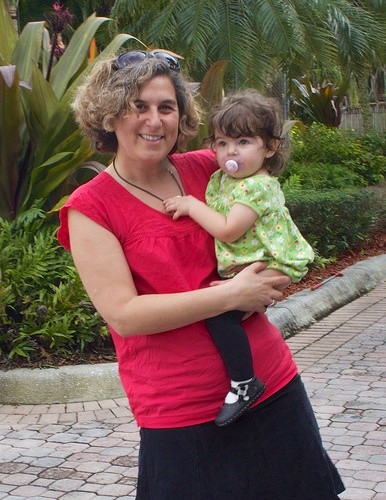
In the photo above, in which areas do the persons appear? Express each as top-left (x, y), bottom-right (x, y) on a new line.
top-left (59, 47), bottom-right (345, 500)
top-left (163, 88), bottom-right (314, 426)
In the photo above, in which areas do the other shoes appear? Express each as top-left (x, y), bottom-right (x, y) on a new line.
top-left (215, 376), bottom-right (265, 428)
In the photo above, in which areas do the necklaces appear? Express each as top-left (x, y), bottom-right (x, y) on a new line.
top-left (112, 154), bottom-right (183, 202)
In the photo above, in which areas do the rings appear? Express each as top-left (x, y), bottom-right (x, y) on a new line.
top-left (270, 300), bottom-right (277, 307)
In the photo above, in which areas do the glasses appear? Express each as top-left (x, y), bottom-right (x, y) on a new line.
top-left (113, 49), bottom-right (181, 72)
